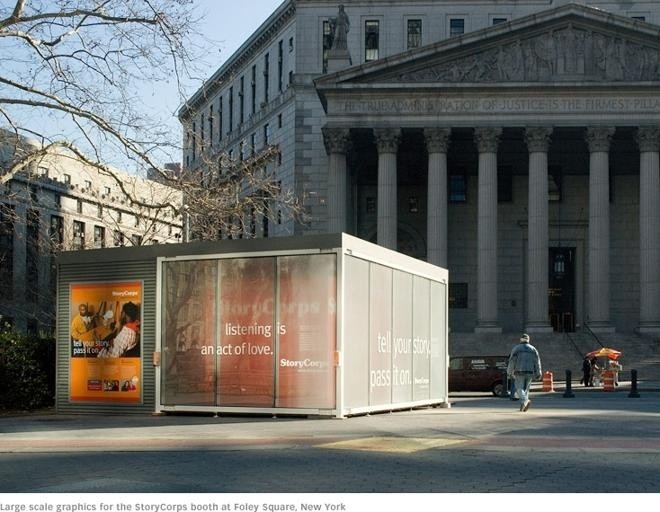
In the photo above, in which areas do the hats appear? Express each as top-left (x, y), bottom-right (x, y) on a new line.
top-left (520, 334), bottom-right (530, 342)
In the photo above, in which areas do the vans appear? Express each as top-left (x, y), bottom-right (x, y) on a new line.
top-left (449, 356), bottom-right (511, 397)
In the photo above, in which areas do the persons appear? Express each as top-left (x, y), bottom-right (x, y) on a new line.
top-left (428, 19), bottom-right (660, 83)
top-left (580, 357), bottom-right (601, 386)
top-left (71, 303), bottom-right (97, 342)
top-left (506, 333), bottom-right (543, 413)
top-left (583, 356), bottom-right (592, 387)
top-left (94, 301), bottom-right (140, 358)
top-left (330, 4), bottom-right (351, 51)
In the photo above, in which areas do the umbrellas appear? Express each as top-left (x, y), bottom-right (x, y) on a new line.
top-left (584, 346), bottom-right (621, 368)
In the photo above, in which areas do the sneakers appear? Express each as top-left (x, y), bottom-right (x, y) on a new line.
top-left (520, 399), bottom-right (530, 411)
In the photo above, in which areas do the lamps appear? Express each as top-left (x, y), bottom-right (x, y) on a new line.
top-left (553, 160), bottom-right (567, 281)
